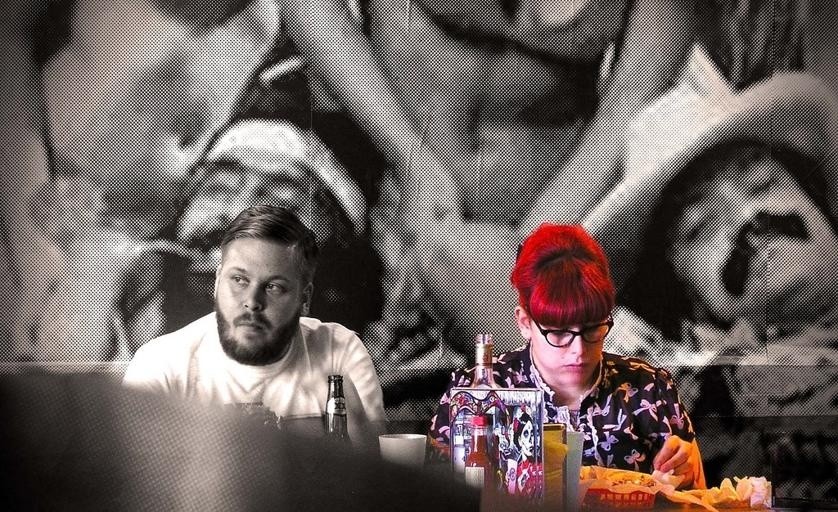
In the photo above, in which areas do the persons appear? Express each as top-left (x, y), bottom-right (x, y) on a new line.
top-left (623, 91), bottom-right (838, 413)
top-left (105, 96), bottom-right (386, 366)
top-left (272, 0), bottom-right (724, 367)
top-left (424, 219), bottom-right (708, 511)
top-left (119, 204), bottom-right (390, 463)
top-left (510, 403), bottom-right (537, 504)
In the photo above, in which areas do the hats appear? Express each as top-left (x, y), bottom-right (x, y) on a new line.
top-left (586, 72), bottom-right (837, 310)
top-left (207, 120), bottom-right (367, 238)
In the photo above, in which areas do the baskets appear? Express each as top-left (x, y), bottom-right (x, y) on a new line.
top-left (581, 488), bottom-right (656, 512)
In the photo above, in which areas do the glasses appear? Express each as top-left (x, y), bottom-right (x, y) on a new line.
top-left (528, 312), bottom-right (614, 347)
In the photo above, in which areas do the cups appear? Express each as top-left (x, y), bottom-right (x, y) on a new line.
top-left (377, 431), bottom-right (428, 475)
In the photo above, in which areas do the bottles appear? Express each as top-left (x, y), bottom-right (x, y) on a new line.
top-left (462, 414), bottom-right (496, 511)
top-left (320, 373), bottom-right (355, 460)
top-left (470, 332), bottom-right (497, 391)
